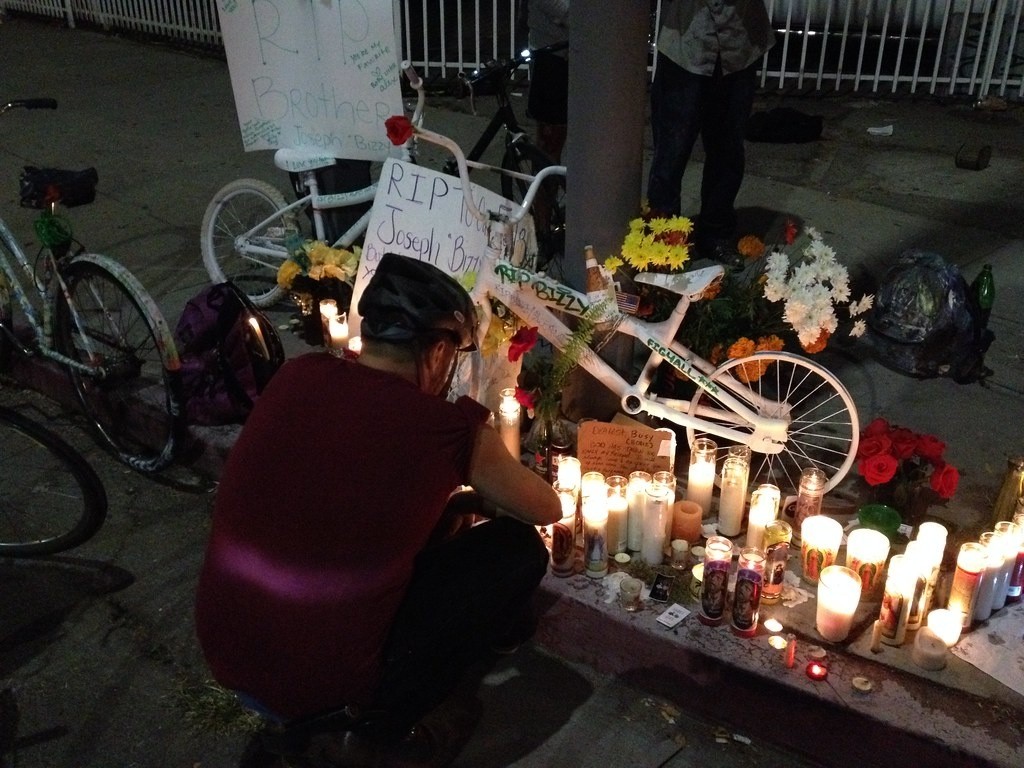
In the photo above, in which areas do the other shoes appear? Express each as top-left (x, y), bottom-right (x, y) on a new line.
top-left (261, 692), bottom-right (483, 768)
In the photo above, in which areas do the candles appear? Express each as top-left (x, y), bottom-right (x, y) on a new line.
top-left (496, 388), bottom-right (1024, 680)
top-left (317, 297), bottom-right (363, 352)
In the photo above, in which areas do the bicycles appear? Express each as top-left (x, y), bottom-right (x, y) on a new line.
top-left (196, 55), bottom-right (429, 308)
top-left (0, 405), bottom-right (110, 559)
top-left (0, 99), bottom-right (190, 474)
top-left (404, 123), bottom-right (862, 506)
top-left (282, 49), bottom-right (568, 269)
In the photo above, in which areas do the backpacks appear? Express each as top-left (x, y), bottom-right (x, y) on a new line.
top-left (163, 281), bottom-right (285, 425)
top-left (862, 249), bottom-right (983, 384)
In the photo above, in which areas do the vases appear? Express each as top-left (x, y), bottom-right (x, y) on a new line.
top-left (893, 476), bottom-right (933, 542)
top-left (288, 274), bottom-right (355, 342)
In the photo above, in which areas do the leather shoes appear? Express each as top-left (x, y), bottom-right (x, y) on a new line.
top-left (698, 237), bottom-right (745, 269)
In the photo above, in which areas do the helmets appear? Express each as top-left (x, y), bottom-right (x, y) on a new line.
top-left (358, 253), bottom-right (478, 349)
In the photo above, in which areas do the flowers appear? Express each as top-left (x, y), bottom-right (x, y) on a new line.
top-left (854, 412), bottom-right (967, 504)
top-left (385, 110), bottom-right (414, 144)
top-left (275, 238), bottom-right (361, 298)
top-left (458, 215), bottom-right (876, 377)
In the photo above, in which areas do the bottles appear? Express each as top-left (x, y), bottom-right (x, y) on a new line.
top-left (970, 263), bottom-right (995, 331)
top-left (584, 244), bottom-right (619, 331)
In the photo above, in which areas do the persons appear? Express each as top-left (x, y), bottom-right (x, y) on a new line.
top-left (643, 0), bottom-right (780, 267)
top-left (526, 0), bottom-right (571, 260)
top-left (188, 253), bottom-right (567, 767)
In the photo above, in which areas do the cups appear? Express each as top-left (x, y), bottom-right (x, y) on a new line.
top-left (857, 503), bottom-right (901, 546)
top-left (620, 578), bottom-right (642, 611)
